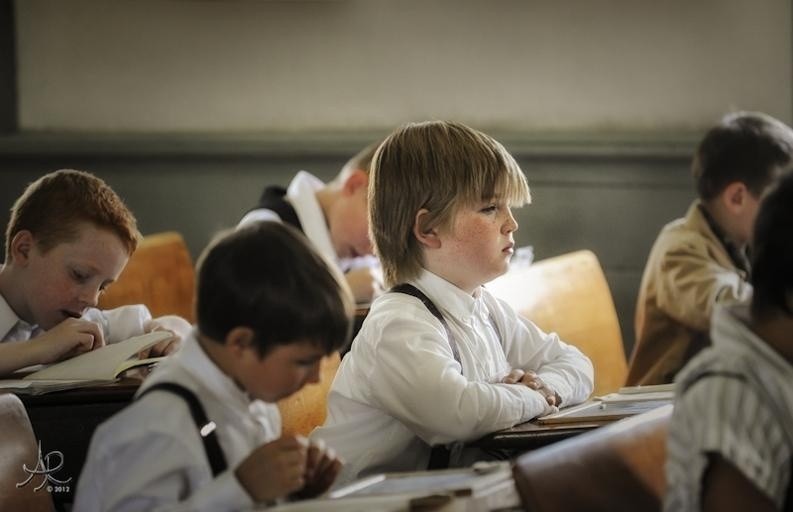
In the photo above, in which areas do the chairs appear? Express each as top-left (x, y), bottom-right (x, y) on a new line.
top-left (0, 228), bottom-right (682, 512)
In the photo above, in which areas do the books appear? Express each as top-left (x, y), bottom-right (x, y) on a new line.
top-left (0, 329), bottom-right (175, 397)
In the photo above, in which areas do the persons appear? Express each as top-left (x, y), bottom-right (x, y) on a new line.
top-left (660, 166), bottom-right (793, 511)
top-left (235, 138), bottom-right (395, 304)
top-left (70, 219), bottom-right (359, 512)
top-left (306, 118), bottom-right (596, 490)
top-left (622, 109), bottom-right (793, 387)
top-left (0, 168), bottom-right (193, 380)
